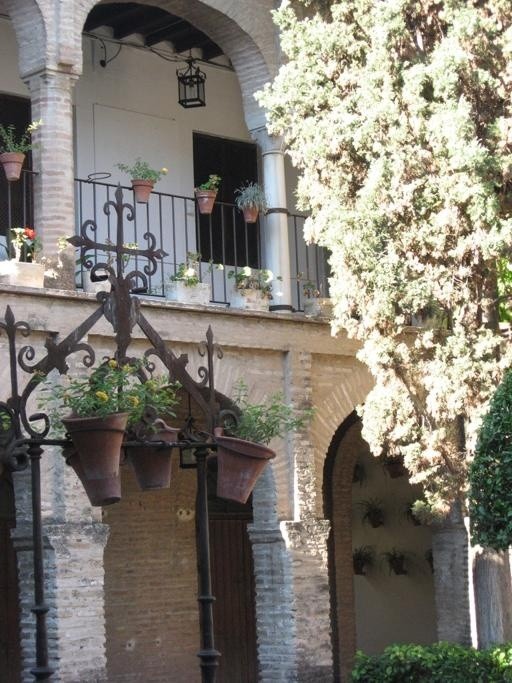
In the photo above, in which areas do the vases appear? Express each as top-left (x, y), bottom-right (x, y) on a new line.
top-left (303, 298), bottom-right (331, 314)
top-left (0, 151), bottom-right (26, 181)
top-left (81, 269), bottom-right (111, 295)
top-left (240, 288), bottom-right (270, 313)
top-left (0, 260), bottom-right (45, 287)
top-left (165, 280), bottom-right (210, 305)
top-left (131, 179), bottom-right (153, 203)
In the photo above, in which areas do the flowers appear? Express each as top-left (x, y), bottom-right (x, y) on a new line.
top-left (170, 250), bottom-right (202, 287)
top-left (235, 265), bottom-right (274, 299)
top-left (299, 273), bottom-right (333, 298)
top-left (116, 156), bottom-right (168, 183)
top-left (33, 359), bottom-right (182, 505)
top-left (7, 227), bottom-right (41, 262)
top-left (0, 119), bottom-right (43, 152)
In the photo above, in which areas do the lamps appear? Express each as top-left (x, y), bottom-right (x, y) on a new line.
top-left (175, 49), bottom-right (206, 108)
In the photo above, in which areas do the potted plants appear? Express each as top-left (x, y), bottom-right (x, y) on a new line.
top-left (234, 179), bottom-right (267, 223)
top-left (194, 174), bottom-right (222, 214)
top-left (351, 454), bottom-right (433, 575)
top-left (209, 374), bottom-right (317, 505)
